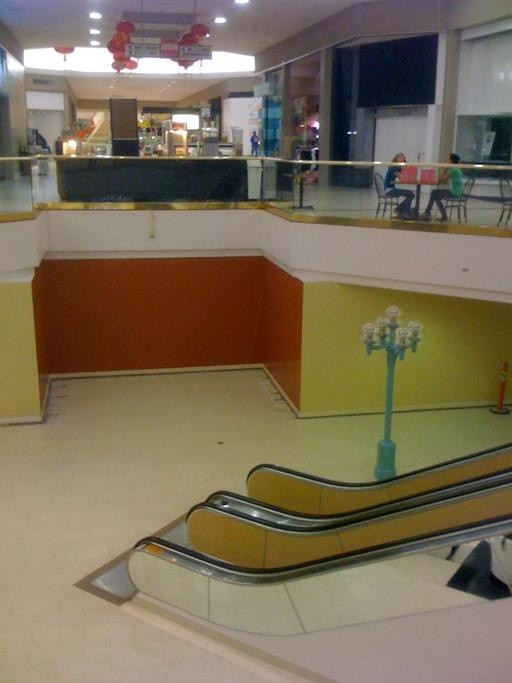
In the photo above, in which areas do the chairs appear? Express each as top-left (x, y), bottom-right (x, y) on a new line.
top-left (373, 171), bottom-right (512, 225)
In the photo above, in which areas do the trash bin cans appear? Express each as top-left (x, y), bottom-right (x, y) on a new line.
top-left (39, 149), bottom-right (48, 175)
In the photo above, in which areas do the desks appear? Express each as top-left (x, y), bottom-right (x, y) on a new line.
top-left (280, 171), bottom-right (317, 213)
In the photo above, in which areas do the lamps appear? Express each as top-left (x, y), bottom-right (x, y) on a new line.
top-left (362, 304), bottom-right (422, 483)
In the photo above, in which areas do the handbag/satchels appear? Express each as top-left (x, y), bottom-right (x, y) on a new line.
top-left (400, 167), bottom-right (435, 183)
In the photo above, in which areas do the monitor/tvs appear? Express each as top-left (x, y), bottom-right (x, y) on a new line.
top-left (356, 33), bottom-right (439, 109)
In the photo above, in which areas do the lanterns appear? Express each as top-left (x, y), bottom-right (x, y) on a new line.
top-left (55, 46), bottom-right (74, 61)
top-left (107, 18), bottom-right (210, 72)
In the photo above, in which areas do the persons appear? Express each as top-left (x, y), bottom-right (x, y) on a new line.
top-left (36, 142), bottom-right (50, 176)
top-left (251, 130), bottom-right (259, 156)
top-left (420, 152), bottom-right (463, 220)
top-left (383, 152), bottom-right (415, 218)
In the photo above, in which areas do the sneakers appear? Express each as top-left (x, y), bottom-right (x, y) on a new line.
top-left (393, 207), bottom-right (448, 221)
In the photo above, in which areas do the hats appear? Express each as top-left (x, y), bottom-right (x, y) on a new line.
top-left (449, 153), bottom-right (461, 163)
